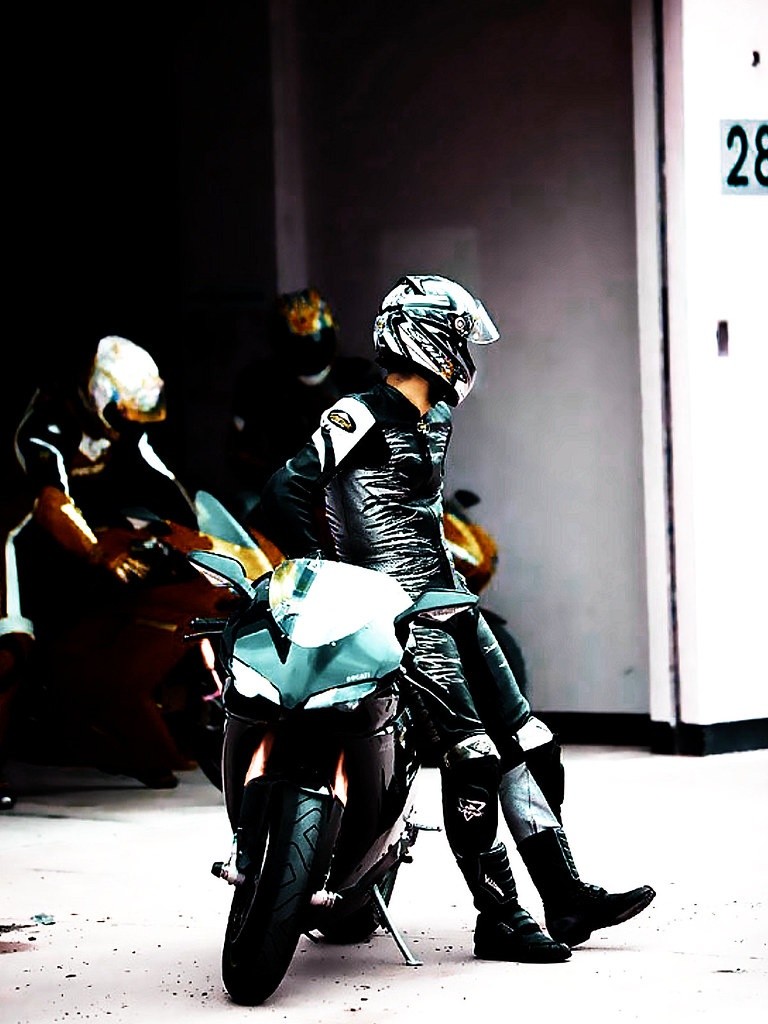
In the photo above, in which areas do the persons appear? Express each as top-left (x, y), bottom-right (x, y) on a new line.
top-left (0, 333), bottom-right (200, 813)
top-left (201, 287), bottom-right (387, 486)
top-left (262, 273), bottom-right (657, 963)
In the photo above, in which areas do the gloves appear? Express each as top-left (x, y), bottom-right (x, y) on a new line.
top-left (92, 540), bottom-right (153, 584)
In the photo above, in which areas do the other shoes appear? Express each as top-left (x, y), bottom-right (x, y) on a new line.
top-left (101, 745), bottom-right (179, 790)
top-left (0, 787), bottom-right (16, 810)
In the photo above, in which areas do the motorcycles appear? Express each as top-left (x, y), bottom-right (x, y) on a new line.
top-left (183, 548), bottom-right (482, 1008)
top-left (242, 488), bottom-right (529, 742)
top-left (40, 493), bottom-right (284, 798)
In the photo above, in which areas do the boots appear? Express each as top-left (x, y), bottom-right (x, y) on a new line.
top-left (515, 827), bottom-right (656, 946)
top-left (456, 842), bottom-right (571, 963)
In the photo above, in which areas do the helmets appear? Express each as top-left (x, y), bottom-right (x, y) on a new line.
top-left (373, 272), bottom-right (478, 410)
top-left (86, 334), bottom-right (164, 430)
top-left (280, 288), bottom-right (337, 375)
